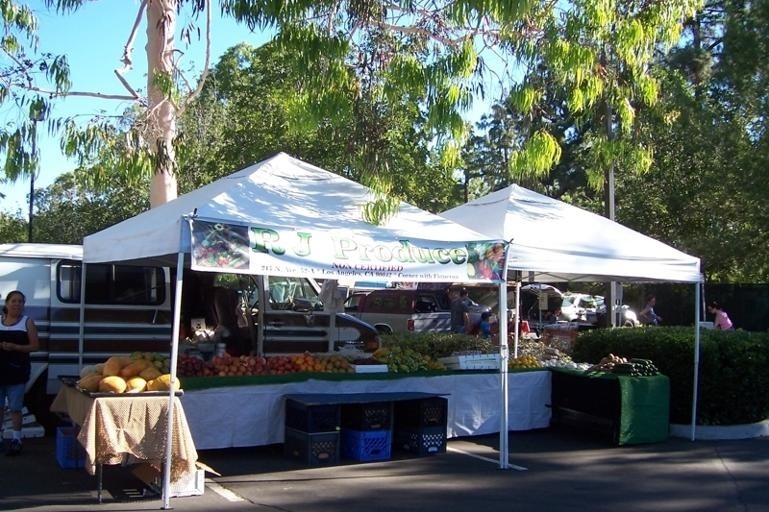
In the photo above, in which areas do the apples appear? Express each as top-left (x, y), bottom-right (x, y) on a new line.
top-left (132, 351), bottom-right (357, 376)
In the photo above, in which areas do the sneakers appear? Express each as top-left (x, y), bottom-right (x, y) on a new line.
top-left (6, 439), bottom-right (24, 456)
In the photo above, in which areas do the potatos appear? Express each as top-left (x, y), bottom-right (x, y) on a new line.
top-left (588, 354), bottom-right (628, 373)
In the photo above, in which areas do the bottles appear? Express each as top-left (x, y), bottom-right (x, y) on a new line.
top-left (216, 343), bottom-right (226, 359)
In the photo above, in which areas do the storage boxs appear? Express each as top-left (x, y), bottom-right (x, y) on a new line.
top-left (43, 436), bottom-right (62, 466)
top-left (436, 354), bottom-right (501, 372)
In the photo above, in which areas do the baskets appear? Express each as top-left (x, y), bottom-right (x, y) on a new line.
top-left (285, 426), bottom-right (340, 467)
top-left (55, 426), bottom-right (85, 469)
top-left (342, 401), bottom-right (391, 463)
top-left (285, 399), bottom-right (341, 433)
top-left (391, 397), bottom-right (448, 459)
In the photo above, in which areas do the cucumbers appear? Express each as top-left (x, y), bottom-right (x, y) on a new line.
top-left (614, 358), bottom-right (659, 377)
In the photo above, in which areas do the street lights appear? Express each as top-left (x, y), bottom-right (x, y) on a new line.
top-left (26, 99), bottom-right (46, 242)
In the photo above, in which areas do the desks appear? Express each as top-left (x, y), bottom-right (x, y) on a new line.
top-left (58, 367), bottom-right (670, 504)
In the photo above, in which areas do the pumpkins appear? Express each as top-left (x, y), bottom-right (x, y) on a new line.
top-left (78, 356), bottom-right (181, 393)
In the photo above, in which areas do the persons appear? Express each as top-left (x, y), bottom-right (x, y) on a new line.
top-left (461, 286), bottom-right (479, 308)
top-left (480, 310), bottom-right (494, 337)
top-left (447, 284), bottom-right (470, 334)
top-left (707, 300), bottom-right (734, 330)
top-left (194, 238), bottom-right (251, 269)
top-left (480, 241), bottom-right (505, 280)
top-left (0, 290), bottom-right (41, 457)
top-left (548, 306), bottom-right (570, 325)
top-left (509, 316), bottom-right (531, 333)
top-left (639, 294), bottom-right (665, 326)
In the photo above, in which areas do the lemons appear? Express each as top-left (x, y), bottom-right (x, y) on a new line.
top-left (508, 354), bottom-right (542, 369)
